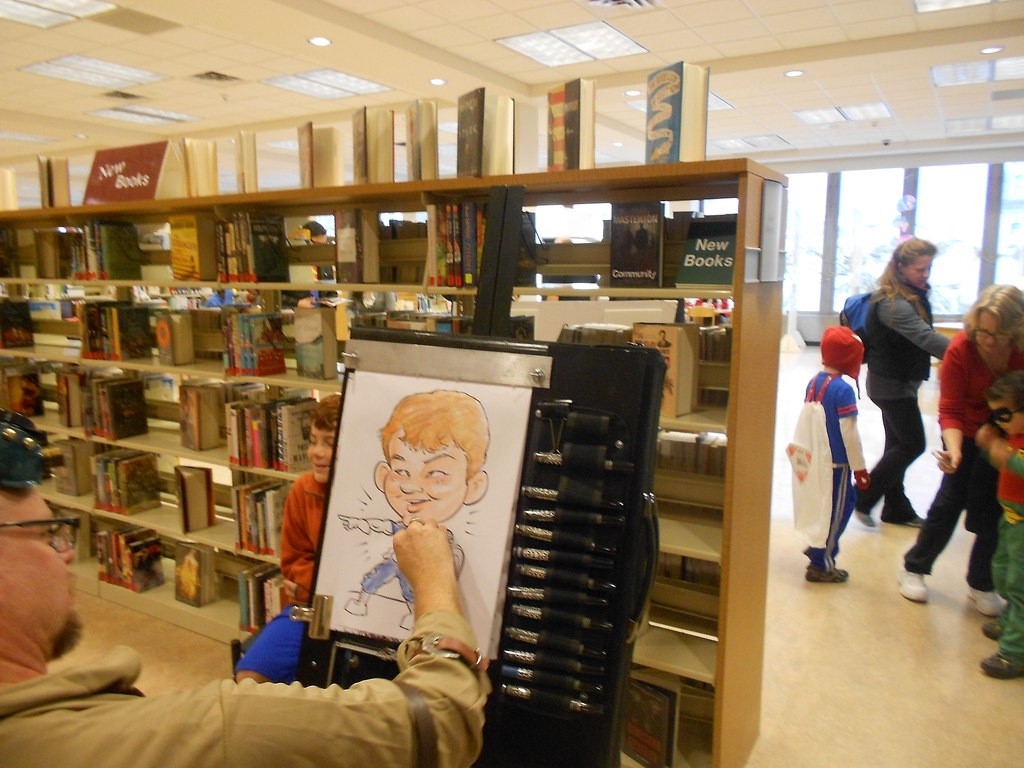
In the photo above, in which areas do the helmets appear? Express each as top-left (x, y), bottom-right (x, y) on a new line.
top-left (0, 407), bottom-right (43, 489)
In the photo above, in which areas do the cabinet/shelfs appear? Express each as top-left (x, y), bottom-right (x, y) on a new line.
top-left (0, 160), bottom-right (790, 768)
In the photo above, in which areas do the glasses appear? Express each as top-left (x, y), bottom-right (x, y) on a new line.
top-left (974, 329), bottom-right (1014, 342)
top-left (0, 518), bottom-right (79, 552)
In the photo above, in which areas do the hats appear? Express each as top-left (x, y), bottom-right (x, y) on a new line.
top-left (821, 325), bottom-right (864, 400)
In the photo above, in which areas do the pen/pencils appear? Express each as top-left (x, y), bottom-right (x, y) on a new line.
top-left (520, 485), bottom-right (624, 509)
top-left (507, 584), bottom-right (607, 607)
top-left (534, 452), bottom-right (634, 474)
top-left (503, 649), bottom-right (604, 675)
top-left (514, 565), bottom-right (616, 591)
top-left (505, 626), bottom-right (606, 660)
top-left (514, 524), bottom-right (615, 551)
top-left (515, 547), bottom-right (612, 569)
top-left (510, 604), bottom-right (612, 631)
top-left (499, 685), bottom-right (601, 715)
top-left (501, 666), bottom-right (602, 693)
top-left (520, 508), bottom-right (625, 525)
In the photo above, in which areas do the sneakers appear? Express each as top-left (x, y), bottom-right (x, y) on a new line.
top-left (806, 568), bottom-right (848, 583)
top-left (967, 585), bottom-right (1002, 617)
top-left (898, 566), bottom-right (928, 603)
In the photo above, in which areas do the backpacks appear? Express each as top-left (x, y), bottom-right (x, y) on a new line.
top-left (839, 292), bottom-right (874, 364)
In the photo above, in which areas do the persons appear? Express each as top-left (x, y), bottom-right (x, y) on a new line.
top-left (235, 391), bottom-right (374, 690)
top-left (281, 290), bottom-right (317, 308)
top-left (0, 422), bottom-right (490, 768)
top-left (303, 221), bottom-right (335, 278)
top-left (657, 330), bottom-right (671, 348)
top-left (788, 236), bottom-right (1024, 681)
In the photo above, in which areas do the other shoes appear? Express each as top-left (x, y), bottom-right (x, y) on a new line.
top-left (980, 654), bottom-right (1024, 679)
top-left (903, 516), bottom-right (925, 527)
top-left (856, 511), bottom-right (875, 527)
top-left (982, 622), bottom-right (1002, 641)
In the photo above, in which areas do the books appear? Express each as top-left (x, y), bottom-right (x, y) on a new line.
top-left (0, 199), bottom-right (741, 768)
